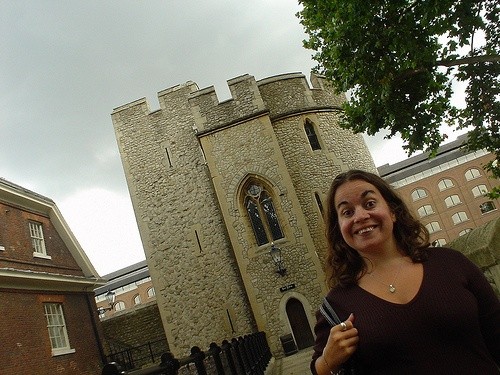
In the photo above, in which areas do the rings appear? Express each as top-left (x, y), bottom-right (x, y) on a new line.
top-left (340, 322), bottom-right (345, 328)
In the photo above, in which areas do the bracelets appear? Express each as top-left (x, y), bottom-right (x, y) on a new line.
top-left (321, 349), bottom-right (332, 373)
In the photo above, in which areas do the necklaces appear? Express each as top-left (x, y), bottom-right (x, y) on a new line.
top-left (362, 257), bottom-right (402, 294)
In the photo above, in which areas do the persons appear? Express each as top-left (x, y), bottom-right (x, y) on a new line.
top-left (310, 171), bottom-right (500, 375)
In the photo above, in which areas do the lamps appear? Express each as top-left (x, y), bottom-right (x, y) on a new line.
top-left (269, 242), bottom-right (286, 276)
